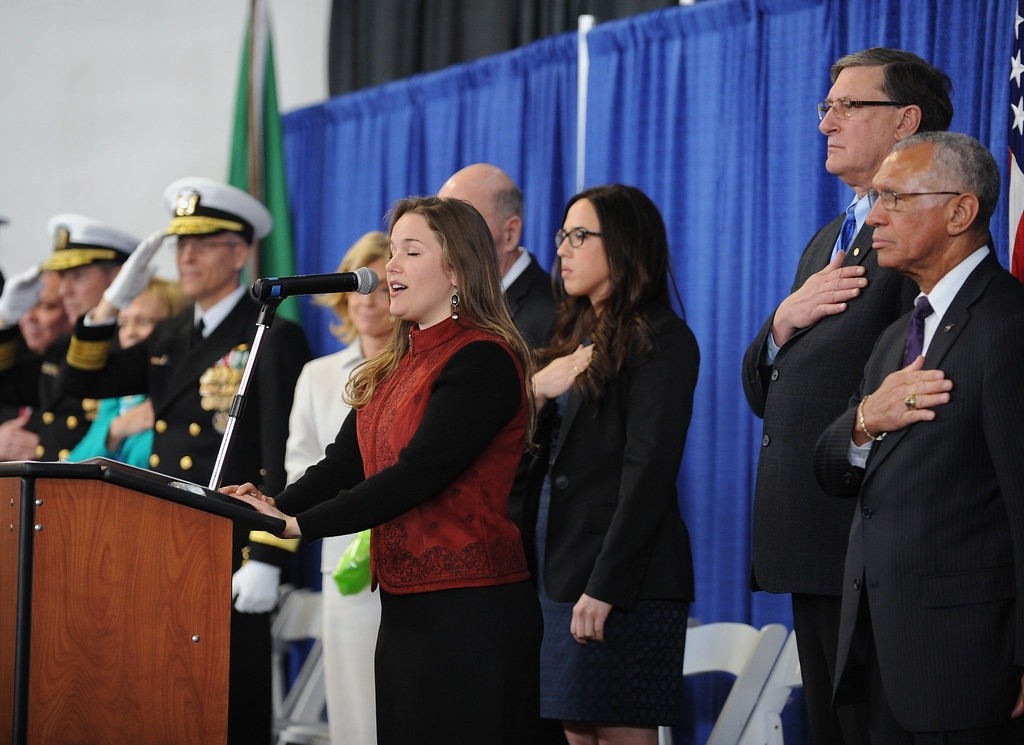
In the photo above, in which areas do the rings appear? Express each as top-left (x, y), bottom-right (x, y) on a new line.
top-left (251, 493), bottom-right (257, 497)
top-left (905, 395), bottom-right (918, 411)
top-left (257, 490), bottom-right (262, 500)
top-left (575, 366), bottom-right (579, 372)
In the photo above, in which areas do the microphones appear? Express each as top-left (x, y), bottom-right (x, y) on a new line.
top-left (254, 268), bottom-right (378, 299)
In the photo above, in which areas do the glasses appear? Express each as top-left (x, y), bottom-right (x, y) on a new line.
top-left (867, 188), bottom-right (965, 210)
top-left (554, 228), bottom-right (608, 249)
top-left (816, 99), bottom-right (906, 122)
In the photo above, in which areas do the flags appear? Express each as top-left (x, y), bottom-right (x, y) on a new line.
top-left (1008, 0), bottom-right (1024, 284)
top-left (227, 0), bottom-right (299, 322)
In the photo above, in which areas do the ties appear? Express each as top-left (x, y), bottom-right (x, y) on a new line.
top-left (836, 203), bottom-right (859, 255)
top-left (195, 317), bottom-right (206, 348)
top-left (903, 295), bottom-right (934, 370)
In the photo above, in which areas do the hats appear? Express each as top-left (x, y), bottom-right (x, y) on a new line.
top-left (39, 215), bottom-right (142, 271)
top-left (165, 178), bottom-right (273, 246)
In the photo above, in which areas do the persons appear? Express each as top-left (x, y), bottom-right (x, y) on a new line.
top-left (741, 47), bottom-right (954, 745)
top-left (529, 184), bottom-right (702, 745)
top-left (284, 231), bottom-right (396, 745)
top-left (814, 131), bottom-right (1024, 745)
top-left (215, 198), bottom-right (545, 745)
top-left (439, 165), bottom-right (570, 745)
top-left (0, 178), bottom-right (305, 745)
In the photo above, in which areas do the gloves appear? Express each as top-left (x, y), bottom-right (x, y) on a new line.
top-left (0, 264), bottom-right (45, 326)
top-left (230, 559), bottom-right (279, 615)
top-left (101, 231), bottom-right (168, 310)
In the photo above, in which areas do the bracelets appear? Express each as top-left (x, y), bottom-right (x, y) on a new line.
top-left (858, 395), bottom-right (887, 441)
